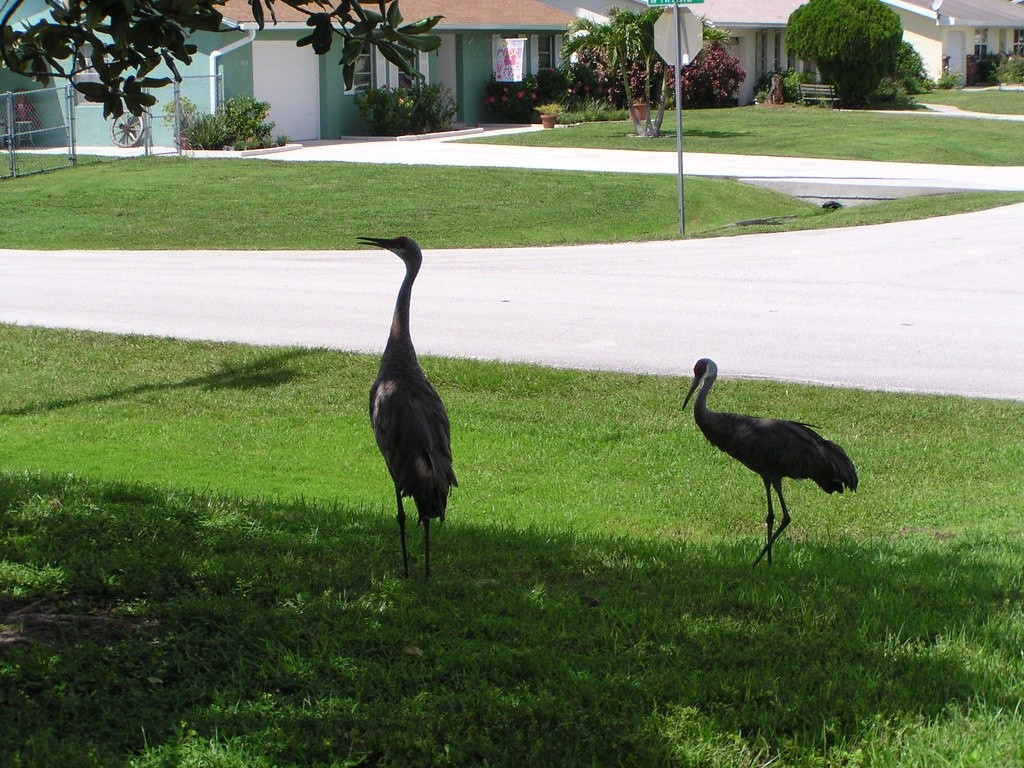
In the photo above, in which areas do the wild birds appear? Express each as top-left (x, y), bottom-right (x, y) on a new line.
top-left (683, 358), bottom-right (858, 567)
top-left (354, 235), bottom-right (458, 578)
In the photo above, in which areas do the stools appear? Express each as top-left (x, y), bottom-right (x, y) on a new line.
top-left (15, 121), bottom-right (36, 148)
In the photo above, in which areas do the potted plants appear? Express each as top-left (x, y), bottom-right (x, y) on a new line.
top-left (533, 102), bottom-right (569, 128)
top-left (626, 97), bottom-right (654, 121)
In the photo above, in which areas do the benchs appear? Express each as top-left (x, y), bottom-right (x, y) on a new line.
top-left (796, 83), bottom-right (843, 108)
